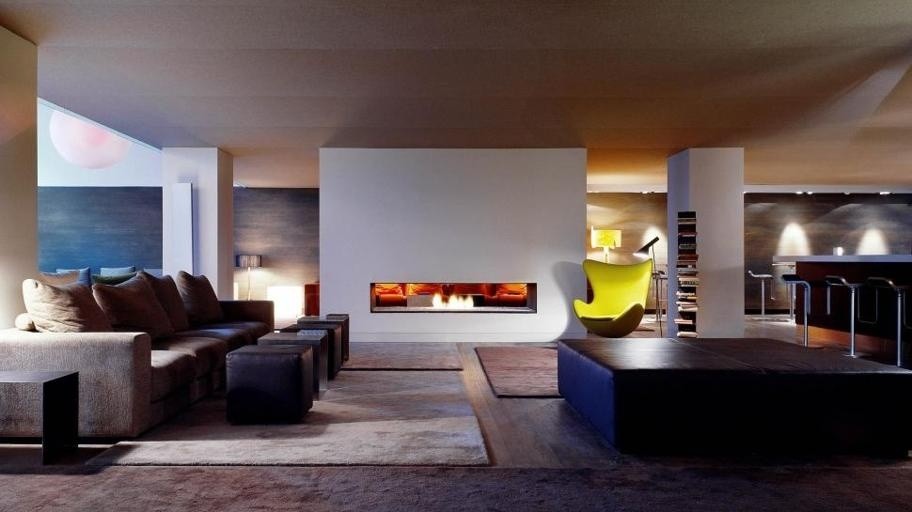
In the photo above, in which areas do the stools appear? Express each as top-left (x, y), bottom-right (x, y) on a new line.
top-left (257, 330), bottom-right (333, 401)
top-left (281, 325), bottom-right (340, 381)
top-left (748, 269), bottom-right (912, 370)
top-left (226, 340), bottom-right (313, 425)
top-left (298, 316), bottom-right (349, 364)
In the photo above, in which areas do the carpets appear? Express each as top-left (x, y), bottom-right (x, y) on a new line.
top-left (344, 340), bottom-right (463, 372)
top-left (0, 463), bottom-right (911, 512)
top-left (475, 346), bottom-right (560, 398)
top-left (83, 372), bottom-right (488, 464)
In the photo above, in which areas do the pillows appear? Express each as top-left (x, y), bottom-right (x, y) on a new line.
top-left (14, 266), bottom-right (222, 339)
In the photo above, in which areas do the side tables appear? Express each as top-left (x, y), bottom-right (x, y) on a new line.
top-left (0, 371), bottom-right (79, 465)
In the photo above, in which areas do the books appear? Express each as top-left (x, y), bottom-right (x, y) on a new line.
top-left (674, 209), bottom-right (701, 338)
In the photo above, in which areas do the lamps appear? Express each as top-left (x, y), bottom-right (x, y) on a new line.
top-left (591, 229), bottom-right (621, 262)
top-left (235, 254), bottom-right (261, 301)
top-left (632, 237), bottom-right (662, 337)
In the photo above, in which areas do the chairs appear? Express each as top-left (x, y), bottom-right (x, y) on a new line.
top-left (573, 259), bottom-right (653, 338)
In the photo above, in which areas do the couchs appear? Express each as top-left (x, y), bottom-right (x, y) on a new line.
top-left (0, 298), bottom-right (275, 437)
